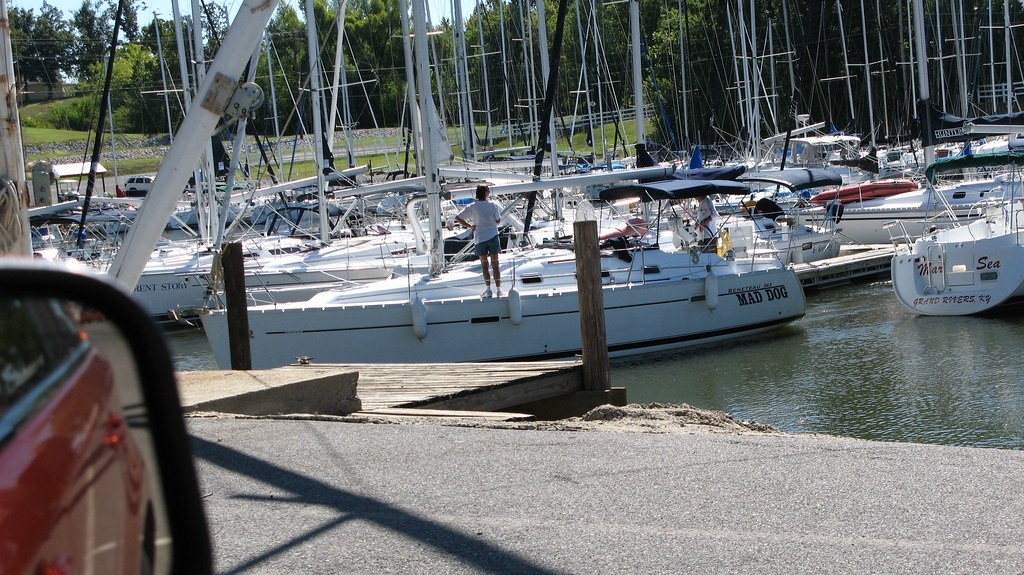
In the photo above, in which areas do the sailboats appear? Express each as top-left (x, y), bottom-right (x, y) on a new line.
top-left (6, 0), bottom-right (1024, 357)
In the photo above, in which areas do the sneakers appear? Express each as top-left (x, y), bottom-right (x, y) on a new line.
top-left (495, 290), bottom-right (507, 299)
top-left (479, 290), bottom-right (491, 297)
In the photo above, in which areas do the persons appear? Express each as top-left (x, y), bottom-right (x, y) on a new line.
top-left (455, 185), bottom-right (506, 298)
top-left (694, 194), bottom-right (721, 253)
top-left (741, 194), bottom-right (756, 213)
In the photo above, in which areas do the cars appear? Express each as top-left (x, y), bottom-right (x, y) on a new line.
top-left (1, 295), bottom-right (161, 575)
